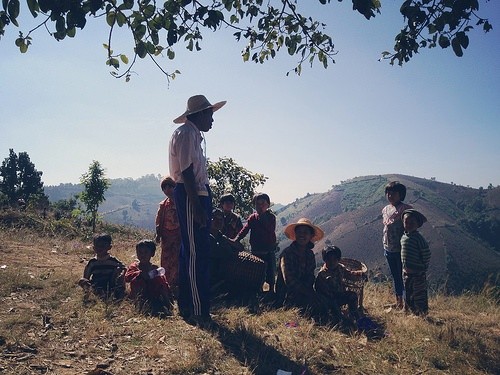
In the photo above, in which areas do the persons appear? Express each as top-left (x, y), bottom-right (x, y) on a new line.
top-left (154, 177), bottom-right (181, 298)
top-left (313, 245), bottom-right (359, 323)
top-left (169, 95), bottom-right (228, 328)
top-left (79, 233), bottom-right (127, 300)
top-left (209, 194), bottom-right (275, 306)
top-left (399, 208), bottom-right (430, 315)
top-left (124, 239), bottom-right (174, 316)
top-left (381, 181), bottom-right (414, 308)
top-left (276, 219), bottom-right (341, 328)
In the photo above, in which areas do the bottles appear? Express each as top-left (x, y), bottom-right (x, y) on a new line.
top-left (147, 266), bottom-right (165, 283)
